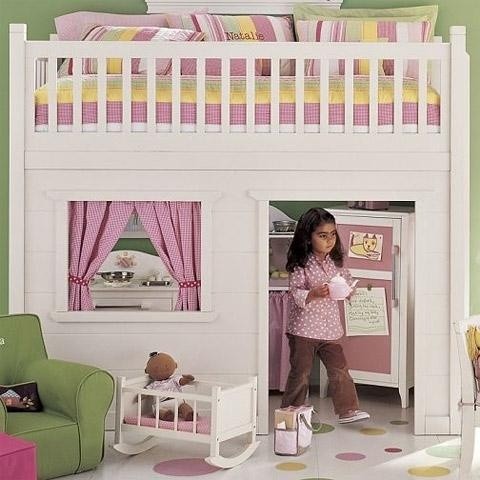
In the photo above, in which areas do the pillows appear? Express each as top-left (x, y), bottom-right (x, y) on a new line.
top-left (53, 9), bottom-right (205, 40)
top-left (295, 4), bottom-right (438, 37)
top-left (164, 14), bottom-right (296, 76)
top-left (59, 25), bottom-right (205, 75)
top-left (294, 15), bottom-right (435, 81)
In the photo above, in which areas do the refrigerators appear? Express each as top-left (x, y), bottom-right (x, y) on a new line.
top-left (325, 204), bottom-right (414, 390)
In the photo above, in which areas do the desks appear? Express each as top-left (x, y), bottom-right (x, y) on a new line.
top-left (88, 250), bottom-right (178, 310)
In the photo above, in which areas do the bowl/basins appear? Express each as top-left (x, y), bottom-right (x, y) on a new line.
top-left (272, 220), bottom-right (296, 232)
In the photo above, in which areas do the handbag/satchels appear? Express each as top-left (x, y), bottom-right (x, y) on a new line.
top-left (275, 406), bottom-right (322, 457)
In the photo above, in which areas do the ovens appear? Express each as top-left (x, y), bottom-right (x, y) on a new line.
top-left (93, 299), bottom-right (144, 311)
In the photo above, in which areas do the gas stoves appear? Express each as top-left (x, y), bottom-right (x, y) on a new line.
top-left (103, 280), bottom-right (132, 287)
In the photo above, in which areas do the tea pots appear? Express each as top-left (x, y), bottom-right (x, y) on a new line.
top-left (321, 273), bottom-right (360, 301)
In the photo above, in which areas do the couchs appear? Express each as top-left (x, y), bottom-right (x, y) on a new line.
top-left (1, 315), bottom-right (116, 480)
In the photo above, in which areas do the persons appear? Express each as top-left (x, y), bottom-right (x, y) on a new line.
top-left (134, 350), bottom-right (196, 423)
top-left (280, 207), bottom-right (371, 425)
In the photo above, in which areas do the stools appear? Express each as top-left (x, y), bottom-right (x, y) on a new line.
top-left (1, 432), bottom-right (38, 479)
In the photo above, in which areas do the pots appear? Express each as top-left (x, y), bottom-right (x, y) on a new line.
top-left (99, 270), bottom-right (135, 283)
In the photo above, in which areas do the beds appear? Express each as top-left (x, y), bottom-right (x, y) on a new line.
top-left (109, 371), bottom-right (261, 468)
top-left (9, 23), bottom-right (471, 437)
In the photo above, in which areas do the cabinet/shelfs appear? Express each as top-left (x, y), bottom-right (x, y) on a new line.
top-left (319, 204), bottom-right (414, 409)
top-left (268, 229), bottom-right (299, 294)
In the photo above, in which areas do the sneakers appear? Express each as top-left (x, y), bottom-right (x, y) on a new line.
top-left (339, 409), bottom-right (371, 424)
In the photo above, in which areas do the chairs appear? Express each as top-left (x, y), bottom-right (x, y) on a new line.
top-left (453, 313), bottom-right (479, 480)
